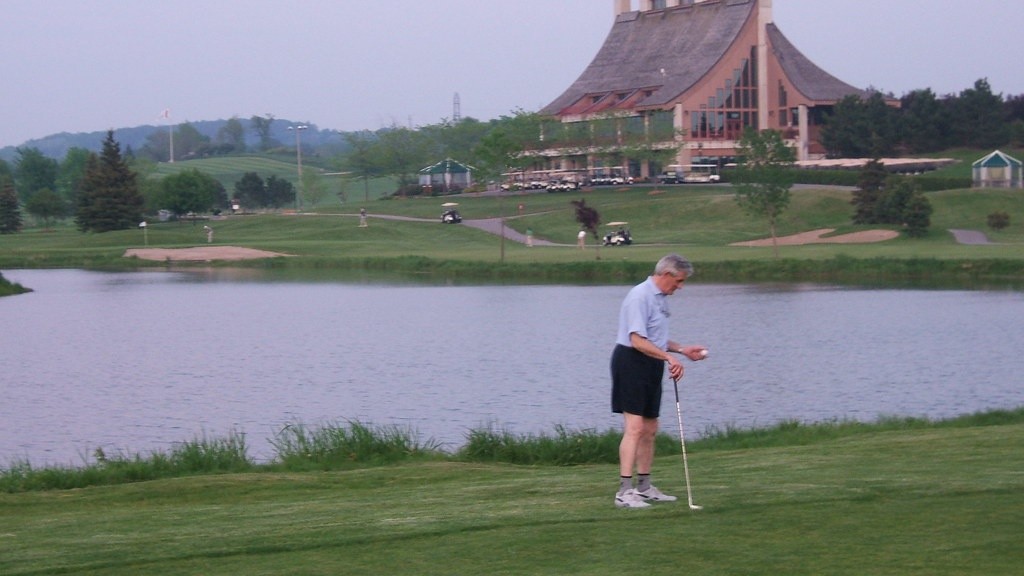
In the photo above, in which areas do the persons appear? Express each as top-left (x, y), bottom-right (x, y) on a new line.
top-left (204, 226), bottom-right (213, 242)
top-left (358, 207), bottom-right (368, 228)
top-left (618, 228), bottom-right (624, 235)
top-left (526, 227), bottom-right (533, 248)
top-left (442, 209), bottom-right (449, 215)
top-left (608, 254), bottom-right (709, 508)
top-left (577, 229), bottom-right (586, 249)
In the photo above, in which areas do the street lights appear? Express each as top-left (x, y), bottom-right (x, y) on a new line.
top-left (286, 124), bottom-right (308, 210)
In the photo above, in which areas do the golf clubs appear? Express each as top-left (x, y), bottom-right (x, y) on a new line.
top-left (674, 377), bottom-right (703, 510)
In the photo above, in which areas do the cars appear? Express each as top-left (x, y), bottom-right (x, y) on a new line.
top-left (499, 177), bottom-right (581, 192)
top-left (657, 170), bottom-right (722, 184)
top-left (589, 173), bottom-right (634, 185)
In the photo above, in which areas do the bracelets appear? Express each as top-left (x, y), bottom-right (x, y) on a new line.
top-left (678, 347), bottom-right (683, 354)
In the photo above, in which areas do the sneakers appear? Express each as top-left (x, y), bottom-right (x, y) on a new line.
top-left (614, 489), bottom-right (652, 508)
top-left (634, 484), bottom-right (678, 501)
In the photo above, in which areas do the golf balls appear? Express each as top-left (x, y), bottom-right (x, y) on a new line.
top-left (701, 349), bottom-right (710, 358)
